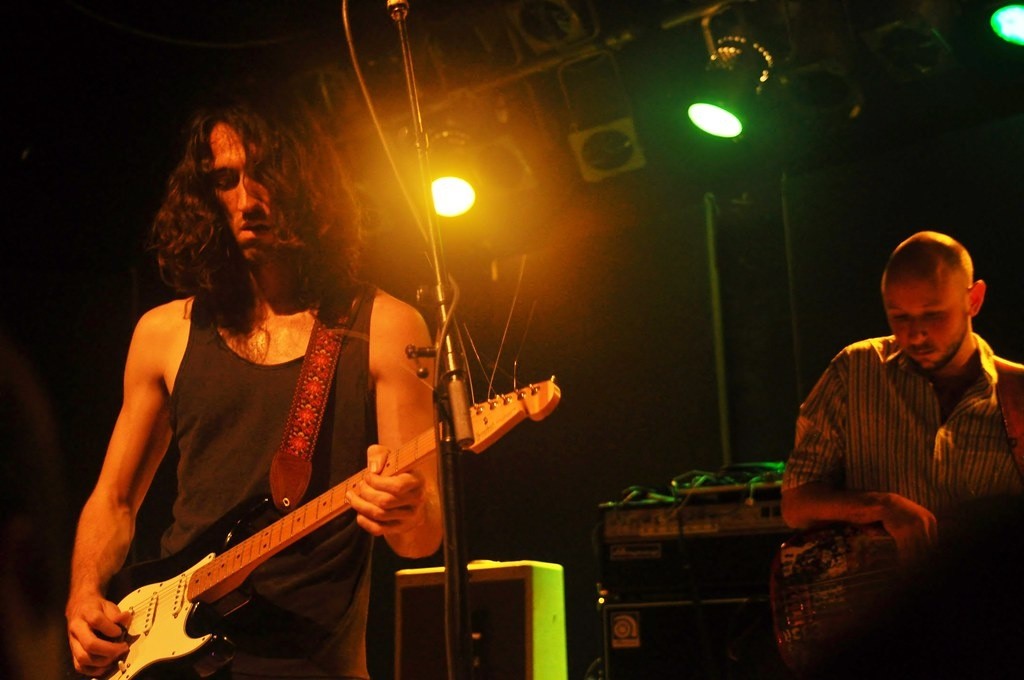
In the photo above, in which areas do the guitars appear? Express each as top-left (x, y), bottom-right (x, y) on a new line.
top-left (769, 483), bottom-right (1024, 679)
top-left (95, 373), bottom-right (560, 679)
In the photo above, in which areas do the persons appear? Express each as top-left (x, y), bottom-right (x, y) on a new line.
top-left (778, 229), bottom-right (1023, 680)
top-left (60, 82), bottom-right (444, 679)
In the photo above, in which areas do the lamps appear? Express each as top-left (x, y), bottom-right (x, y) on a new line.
top-left (688, 22), bottom-right (793, 139)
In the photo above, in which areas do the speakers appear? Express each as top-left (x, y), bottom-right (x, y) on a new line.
top-left (394, 559), bottom-right (568, 680)
